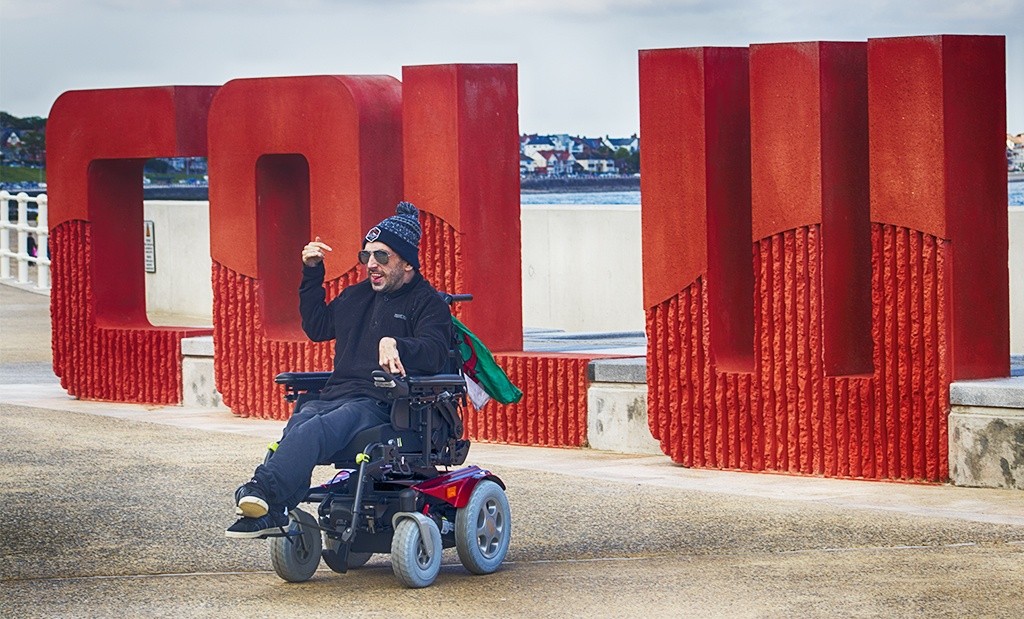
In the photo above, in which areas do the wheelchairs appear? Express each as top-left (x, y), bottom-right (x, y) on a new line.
top-left (220, 290), bottom-right (513, 591)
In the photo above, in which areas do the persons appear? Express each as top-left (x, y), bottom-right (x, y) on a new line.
top-left (223, 202), bottom-right (453, 540)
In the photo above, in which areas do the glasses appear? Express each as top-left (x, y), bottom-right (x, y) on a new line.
top-left (358, 248), bottom-right (396, 266)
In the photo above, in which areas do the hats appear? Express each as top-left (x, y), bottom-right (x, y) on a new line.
top-left (362, 201), bottom-right (423, 269)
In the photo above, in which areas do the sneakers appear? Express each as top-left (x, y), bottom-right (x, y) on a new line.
top-left (234, 481), bottom-right (269, 518)
top-left (226, 508), bottom-right (290, 539)
